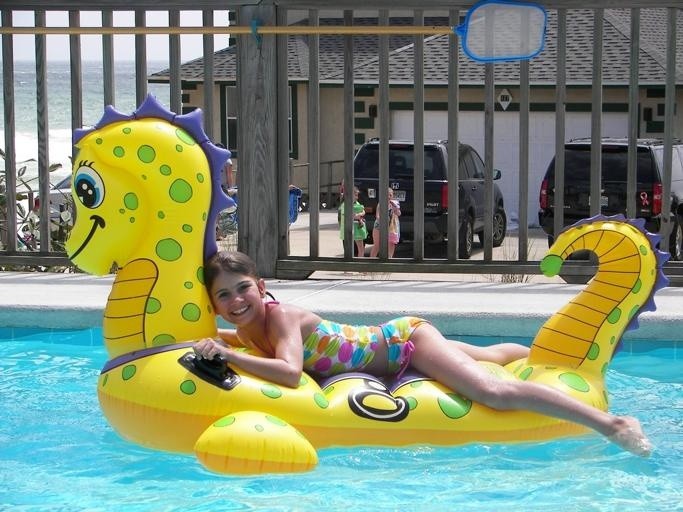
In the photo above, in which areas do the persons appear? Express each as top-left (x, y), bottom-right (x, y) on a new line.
top-left (370, 188), bottom-right (401, 257)
top-left (215, 143), bottom-right (233, 193)
top-left (340, 188), bottom-right (366, 256)
top-left (193, 252), bottom-right (652, 459)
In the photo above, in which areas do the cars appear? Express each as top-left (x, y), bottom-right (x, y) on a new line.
top-left (33, 172), bottom-right (75, 241)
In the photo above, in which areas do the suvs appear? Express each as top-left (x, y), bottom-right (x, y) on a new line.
top-left (535, 136), bottom-right (683, 259)
top-left (335, 137), bottom-right (507, 258)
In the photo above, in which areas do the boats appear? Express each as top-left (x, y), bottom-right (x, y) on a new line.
top-left (48, 96), bottom-right (660, 475)
